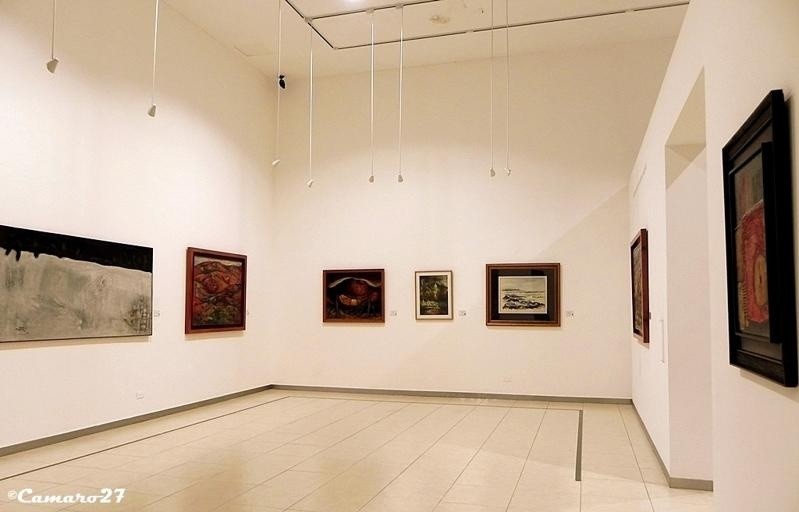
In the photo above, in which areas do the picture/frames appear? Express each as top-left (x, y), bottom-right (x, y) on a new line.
top-left (628, 227), bottom-right (650, 345)
top-left (721, 89), bottom-right (799, 390)
top-left (485, 263), bottom-right (560, 328)
top-left (322, 268), bottom-right (386, 323)
top-left (414, 268), bottom-right (456, 321)
top-left (185, 245), bottom-right (246, 336)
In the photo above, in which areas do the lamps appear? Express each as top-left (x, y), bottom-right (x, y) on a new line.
top-left (306, 21), bottom-right (314, 189)
top-left (46, 1), bottom-right (61, 75)
top-left (147, 1), bottom-right (159, 118)
top-left (367, 13), bottom-right (376, 184)
top-left (397, 6), bottom-right (404, 184)
top-left (503, 1), bottom-right (514, 178)
top-left (271, 1), bottom-right (281, 168)
top-left (488, 1), bottom-right (499, 177)
